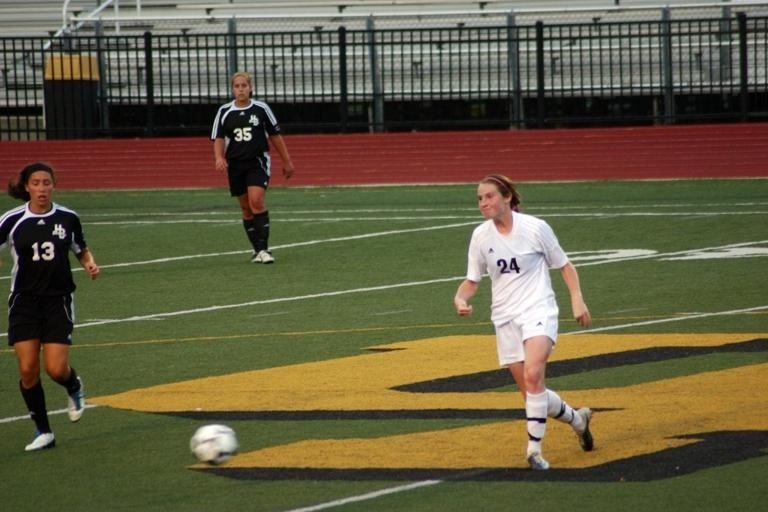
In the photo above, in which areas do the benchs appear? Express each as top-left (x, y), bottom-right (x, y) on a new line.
top-left (0, 0), bottom-right (767, 108)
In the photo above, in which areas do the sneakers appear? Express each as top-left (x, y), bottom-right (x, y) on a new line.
top-left (67, 374), bottom-right (85, 424)
top-left (576, 407), bottom-right (594, 452)
top-left (24, 432), bottom-right (56, 453)
top-left (250, 249), bottom-right (274, 264)
top-left (526, 452), bottom-right (551, 472)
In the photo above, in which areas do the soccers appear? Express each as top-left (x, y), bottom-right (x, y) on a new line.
top-left (190, 425), bottom-right (238, 464)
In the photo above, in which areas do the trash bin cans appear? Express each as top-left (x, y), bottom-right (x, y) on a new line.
top-left (43, 53), bottom-right (101, 140)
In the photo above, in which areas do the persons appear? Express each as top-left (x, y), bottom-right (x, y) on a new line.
top-left (210, 71), bottom-right (293, 262)
top-left (0, 163), bottom-right (99, 452)
top-left (455, 174), bottom-right (593, 469)
